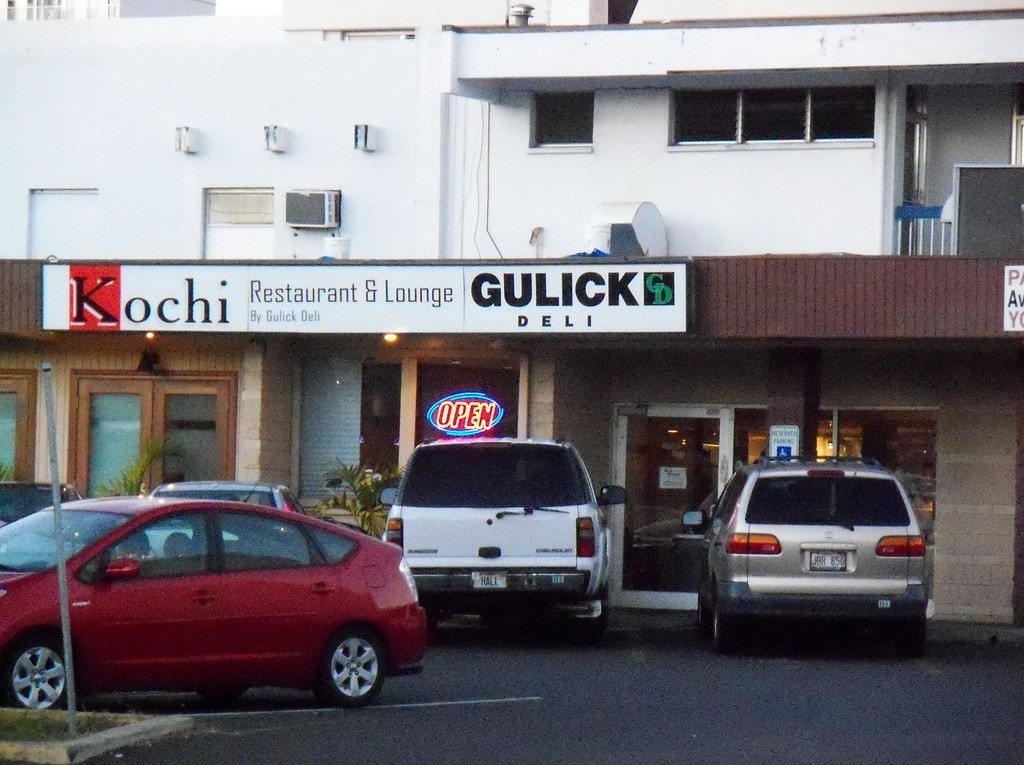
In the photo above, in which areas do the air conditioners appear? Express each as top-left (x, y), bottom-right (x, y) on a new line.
top-left (286, 190), bottom-right (340, 227)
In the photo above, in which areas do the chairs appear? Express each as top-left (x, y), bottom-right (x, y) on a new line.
top-left (836, 491), bottom-right (873, 513)
top-left (760, 488), bottom-right (801, 515)
top-left (121, 529), bottom-right (149, 555)
top-left (164, 532), bottom-right (189, 559)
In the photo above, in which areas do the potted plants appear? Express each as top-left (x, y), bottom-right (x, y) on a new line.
top-left (322, 453), bottom-right (404, 537)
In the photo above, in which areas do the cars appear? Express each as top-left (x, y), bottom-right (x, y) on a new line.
top-left (382, 437), bottom-right (627, 647)
top-left (0, 480), bottom-right (427, 709)
top-left (681, 456), bottom-right (929, 658)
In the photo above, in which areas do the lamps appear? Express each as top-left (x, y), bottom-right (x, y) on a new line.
top-left (136, 342), bottom-right (159, 375)
top-left (510, 4), bottom-right (534, 15)
